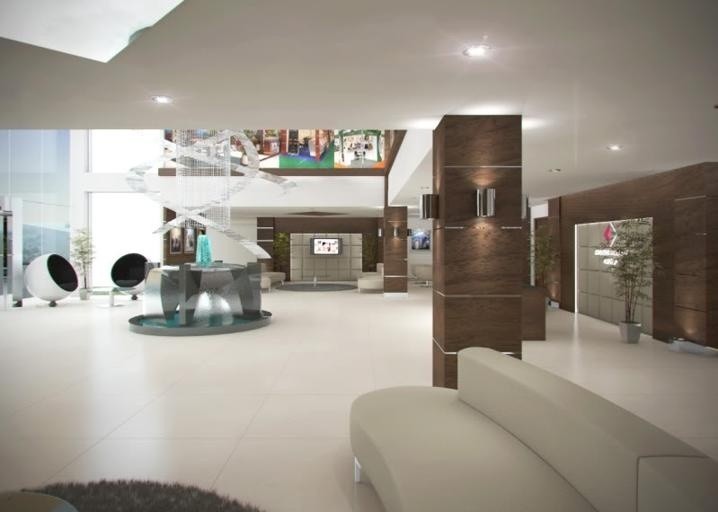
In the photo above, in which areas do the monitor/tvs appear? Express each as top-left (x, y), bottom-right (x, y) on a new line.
top-left (310, 237), bottom-right (342, 256)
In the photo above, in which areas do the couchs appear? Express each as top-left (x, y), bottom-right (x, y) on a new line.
top-left (259, 262), bottom-right (286, 293)
top-left (351, 347), bottom-right (717, 512)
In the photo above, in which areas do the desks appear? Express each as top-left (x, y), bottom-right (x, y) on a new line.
top-left (154, 263), bottom-right (270, 327)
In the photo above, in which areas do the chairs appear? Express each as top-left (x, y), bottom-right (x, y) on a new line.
top-left (357, 263), bottom-right (384, 294)
top-left (23, 253), bottom-right (78, 307)
top-left (110, 253), bottom-right (152, 300)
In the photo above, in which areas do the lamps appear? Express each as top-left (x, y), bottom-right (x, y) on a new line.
top-left (407, 228), bottom-right (412, 237)
top-left (378, 228), bottom-right (383, 237)
top-left (475, 188), bottom-right (497, 218)
top-left (419, 194), bottom-right (438, 219)
top-left (392, 227), bottom-right (399, 237)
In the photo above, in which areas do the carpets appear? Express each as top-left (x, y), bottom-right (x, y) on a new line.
top-left (274, 283), bottom-right (358, 291)
top-left (20, 479), bottom-right (268, 511)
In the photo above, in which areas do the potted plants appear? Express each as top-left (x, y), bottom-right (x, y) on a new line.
top-left (595, 215), bottom-right (675, 344)
top-left (69, 228), bottom-right (96, 300)
top-left (525, 225), bottom-right (560, 309)
